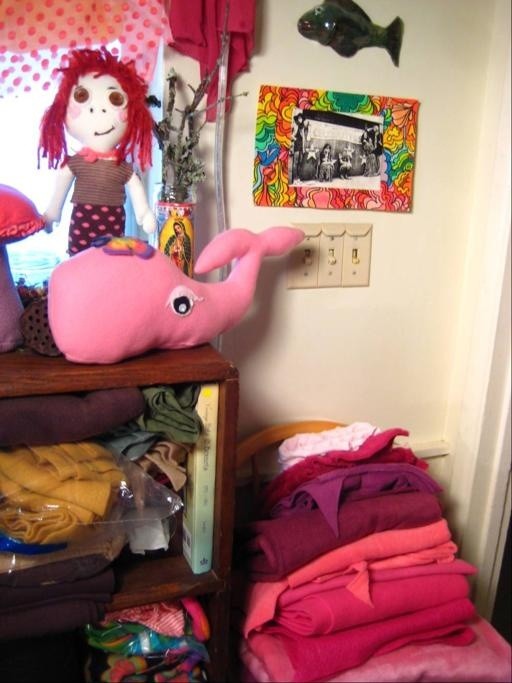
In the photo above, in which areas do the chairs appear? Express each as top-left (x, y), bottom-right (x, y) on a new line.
top-left (233, 418), bottom-right (512, 681)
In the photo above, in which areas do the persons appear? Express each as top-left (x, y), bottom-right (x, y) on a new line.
top-left (163, 219), bottom-right (190, 275)
top-left (291, 112), bottom-right (383, 186)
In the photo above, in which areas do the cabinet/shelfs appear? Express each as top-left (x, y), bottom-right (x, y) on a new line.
top-left (0, 335), bottom-right (243, 681)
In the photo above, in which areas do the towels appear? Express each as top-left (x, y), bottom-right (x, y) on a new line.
top-left (243, 421), bottom-right (479, 681)
top-left (162, 0), bottom-right (261, 130)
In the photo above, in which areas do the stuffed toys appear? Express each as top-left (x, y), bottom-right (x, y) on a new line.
top-left (37, 48), bottom-right (158, 258)
top-left (47, 226), bottom-right (305, 363)
